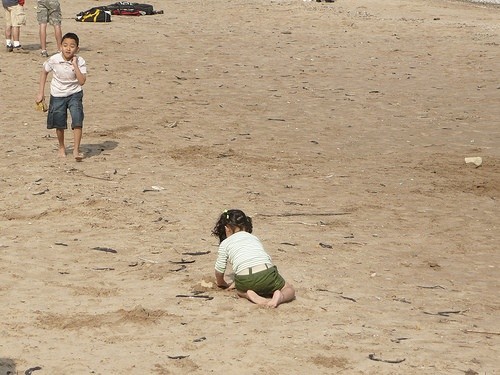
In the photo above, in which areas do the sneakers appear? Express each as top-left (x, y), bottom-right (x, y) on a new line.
top-left (7, 45), bottom-right (13, 51)
top-left (12, 45), bottom-right (29, 53)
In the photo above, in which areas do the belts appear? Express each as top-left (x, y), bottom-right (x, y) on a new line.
top-left (237, 264), bottom-right (272, 275)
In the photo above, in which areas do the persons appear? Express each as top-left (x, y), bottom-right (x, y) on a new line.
top-left (2, 0), bottom-right (28, 53)
top-left (211, 209), bottom-right (295, 309)
top-left (37, 0), bottom-right (63, 56)
top-left (35, 33), bottom-right (87, 162)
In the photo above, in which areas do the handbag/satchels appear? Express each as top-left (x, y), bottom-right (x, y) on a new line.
top-left (77, 8), bottom-right (111, 22)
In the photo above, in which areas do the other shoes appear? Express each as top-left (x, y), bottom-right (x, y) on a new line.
top-left (52, 50), bottom-right (61, 56)
top-left (41, 49), bottom-right (48, 57)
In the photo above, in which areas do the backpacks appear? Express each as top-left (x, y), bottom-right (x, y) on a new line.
top-left (106, 1), bottom-right (153, 16)
